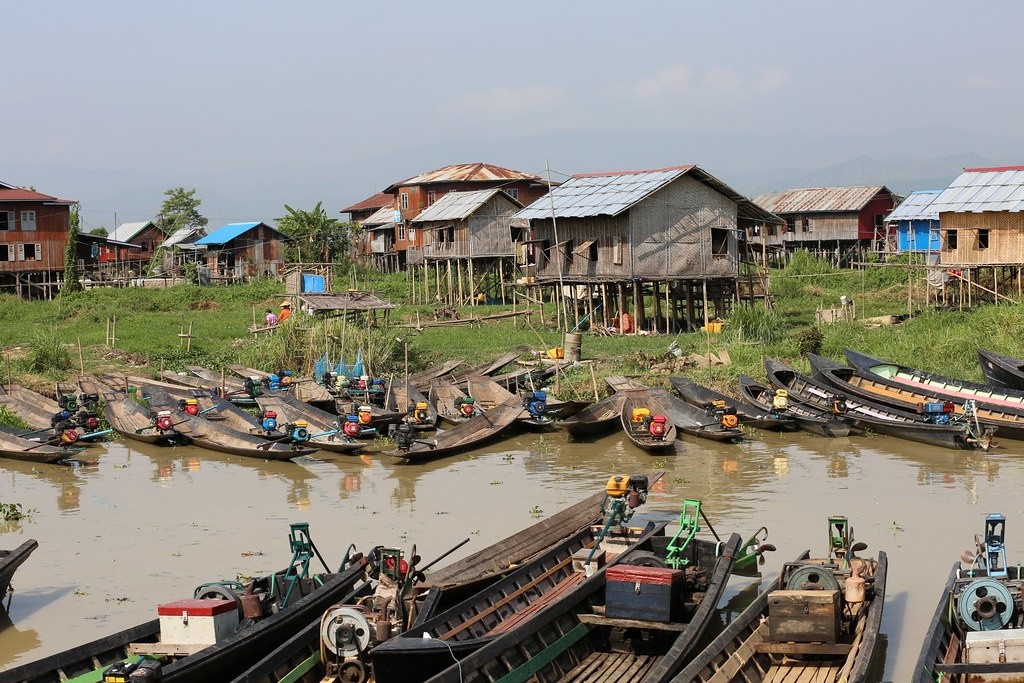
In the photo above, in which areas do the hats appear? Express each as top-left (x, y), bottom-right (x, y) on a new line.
top-left (280, 301), bottom-right (290, 306)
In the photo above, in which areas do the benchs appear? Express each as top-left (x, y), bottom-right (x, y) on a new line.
top-left (755, 634), bottom-right (853, 655)
top-left (577, 605), bottom-right (690, 632)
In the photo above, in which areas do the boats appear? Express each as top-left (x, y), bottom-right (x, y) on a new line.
top-left (0, 344), bottom-right (593, 461)
top-left (664, 515), bottom-right (887, 683)
top-left (974, 349), bottom-right (1024, 390)
top-left (0, 475), bottom-right (778, 683)
top-left (554, 347), bottom-right (1023, 451)
top-left (911, 507), bottom-right (1024, 683)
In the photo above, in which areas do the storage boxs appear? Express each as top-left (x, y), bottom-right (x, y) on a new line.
top-left (965, 628), bottom-right (1024, 683)
top-left (605, 563), bottom-right (685, 624)
top-left (768, 590), bottom-right (843, 645)
top-left (158, 598), bottom-right (240, 644)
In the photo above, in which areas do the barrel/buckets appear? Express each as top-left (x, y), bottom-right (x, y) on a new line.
top-left (564, 332), bottom-right (583, 362)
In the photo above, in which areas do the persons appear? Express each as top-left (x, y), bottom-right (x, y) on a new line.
top-left (272, 301), bottom-right (295, 326)
top-left (265, 308), bottom-right (277, 338)
